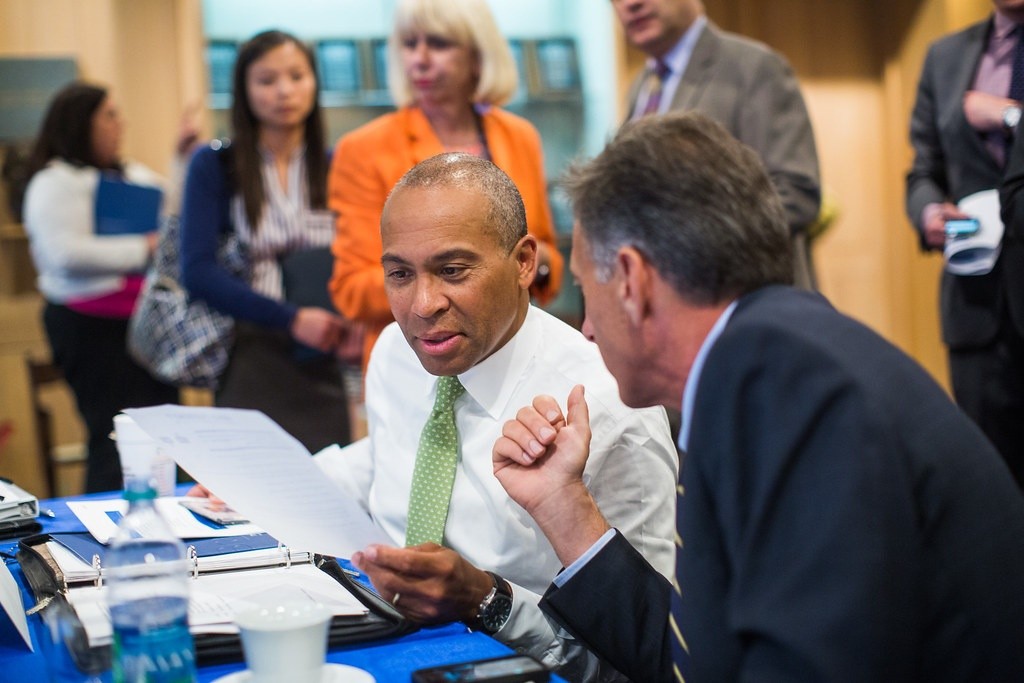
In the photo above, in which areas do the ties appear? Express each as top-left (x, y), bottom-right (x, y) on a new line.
top-left (407, 373), bottom-right (465, 548)
top-left (663, 444), bottom-right (691, 683)
top-left (645, 56), bottom-right (675, 116)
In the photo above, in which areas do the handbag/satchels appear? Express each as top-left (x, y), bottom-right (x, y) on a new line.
top-left (128, 137), bottom-right (245, 387)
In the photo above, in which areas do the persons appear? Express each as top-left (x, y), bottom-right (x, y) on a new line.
top-left (325, 0), bottom-right (563, 400)
top-left (176, 29), bottom-right (365, 456)
top-left (905, 0), bottom-right (1024, 482)
top-left (12, 79), bottom-right (184, 493)
top-left (490, 108), bottom-right (1024, 683)
top-left (185, 152), bottom-right (680, 683)
top-left (607, 0), bottom-right (822, 291)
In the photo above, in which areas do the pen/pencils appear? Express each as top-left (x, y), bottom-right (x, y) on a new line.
top-left (38, 507), bottom-right (55, 520)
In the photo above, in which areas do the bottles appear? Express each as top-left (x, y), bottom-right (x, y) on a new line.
top-left (104, 466), bottom-right (199, 682)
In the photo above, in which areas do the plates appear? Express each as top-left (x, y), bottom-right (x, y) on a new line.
top-left (211, 663), bottom-right (378, 683)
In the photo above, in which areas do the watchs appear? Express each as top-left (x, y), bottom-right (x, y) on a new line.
top-left (1003, 106), bottom-right (1022, 126)
top-left (467, 571), bottom-right (513, 634)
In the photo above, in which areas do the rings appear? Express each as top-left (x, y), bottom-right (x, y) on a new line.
top-left (392, 593), bottom-right (400, 606)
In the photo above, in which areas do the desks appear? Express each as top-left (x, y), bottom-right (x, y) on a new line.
top-left (0, 483), bottom-right (570, 683)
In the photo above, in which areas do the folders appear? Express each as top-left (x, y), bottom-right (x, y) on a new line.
top-left (0, 477), bottom-right (38, 523)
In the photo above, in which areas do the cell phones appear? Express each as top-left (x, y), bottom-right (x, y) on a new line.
top-left (944, 218), bottom-right (980, 238)
top-left (412, 654), bottom-right (548, 683)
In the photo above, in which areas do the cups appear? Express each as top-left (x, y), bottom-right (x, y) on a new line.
top-left (114, 414), bottom-right (175, 497)
top-left (227, 589), bottom-right (333, 683)
